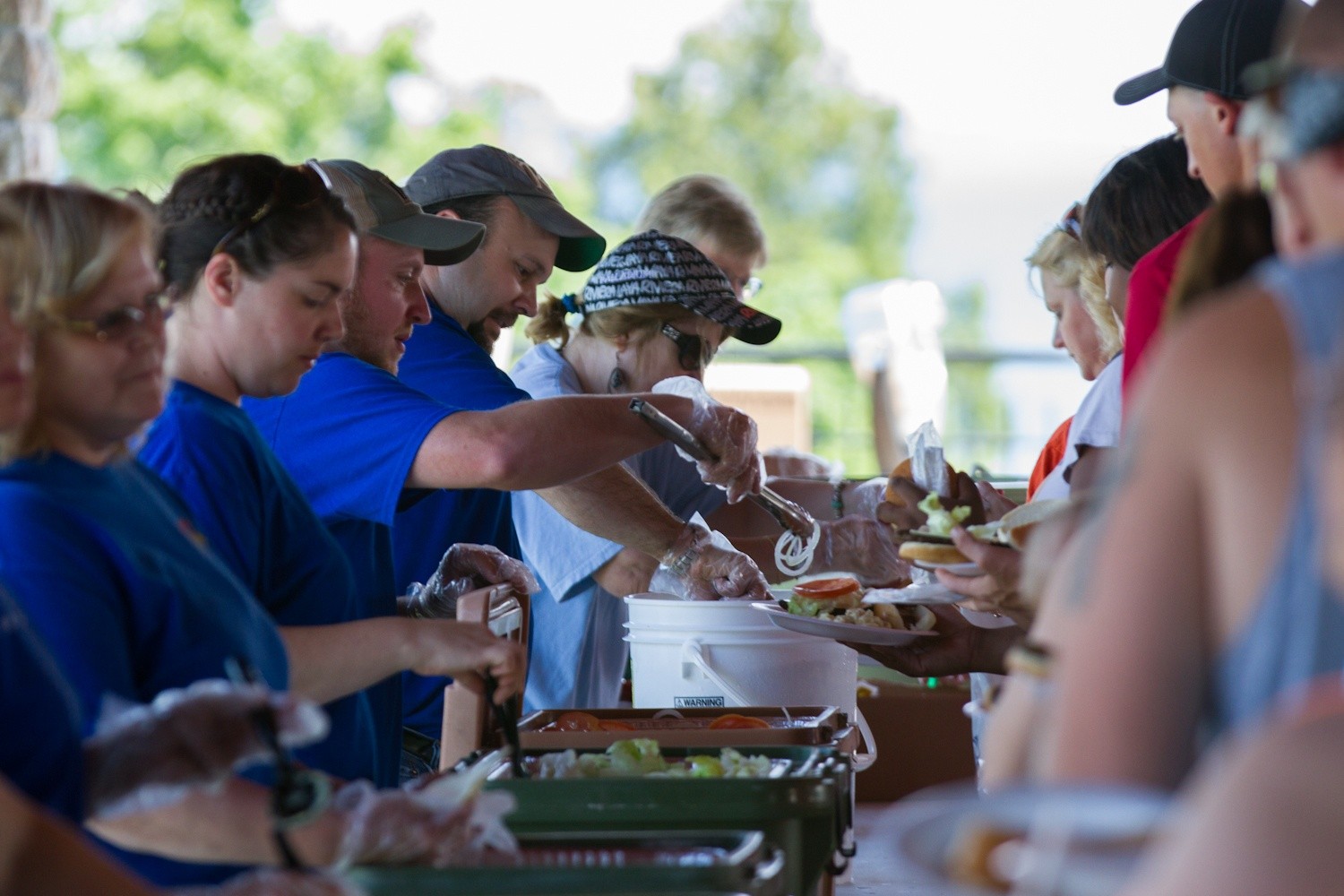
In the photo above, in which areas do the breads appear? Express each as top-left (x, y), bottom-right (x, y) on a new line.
top-left (898, 540), bottom-right (972, 562)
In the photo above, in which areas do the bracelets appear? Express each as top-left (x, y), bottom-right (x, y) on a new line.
top-left (832, 479), bottom-right (850, 517)
top-left (668, 528), bottom-right (704, 578)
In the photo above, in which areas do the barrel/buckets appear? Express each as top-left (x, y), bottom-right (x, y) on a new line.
top-left (623, 593), bottom-right (858, 726)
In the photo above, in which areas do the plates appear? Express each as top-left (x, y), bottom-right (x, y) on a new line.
top-left (914, 560), bottom-right (987, 577)
top-left (751, 602), bottom-right (939, 647)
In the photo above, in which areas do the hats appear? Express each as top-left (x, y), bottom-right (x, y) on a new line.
top-left (582, 230), bottom-right (781, 345)
top-left (1114, 0), bottom-right (1289, 105)
top-left (404, 144), bottom-right (606, 272)
top-left (306, 157), bottom-right (485, 267)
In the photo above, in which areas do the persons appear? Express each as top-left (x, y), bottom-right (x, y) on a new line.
top-left (1, 0), bottom-right (1344, 896)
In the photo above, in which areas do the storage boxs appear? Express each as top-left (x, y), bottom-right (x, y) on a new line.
top-left (349, 699), bottom-right (863, 896)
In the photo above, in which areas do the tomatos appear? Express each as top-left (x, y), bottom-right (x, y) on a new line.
top-left (792, 578), bottom-right (860, 599)
top-left (542, 710), bottom-right (636, 731)
top-left (706, 714), bottom-right (769, 728)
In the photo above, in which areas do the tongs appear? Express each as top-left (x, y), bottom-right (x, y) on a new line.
top-left (629, 398), bottom-right (815, 544)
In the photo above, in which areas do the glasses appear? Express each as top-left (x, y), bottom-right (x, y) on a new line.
top-left (210, 164), bottom-right (321, 259)
top-left (660, 325), bottom-right (716, 371)
top-left (1057, 202), bottom-right (1086, 241)
top-left (62, 291), bottom-right (173, 345)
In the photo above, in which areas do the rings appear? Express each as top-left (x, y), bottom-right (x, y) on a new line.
top-left (993, 612), bottom-right (1002, 619)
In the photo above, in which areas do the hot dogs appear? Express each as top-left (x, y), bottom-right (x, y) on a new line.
top-left (870, 602), bottom-right (936, 631)
